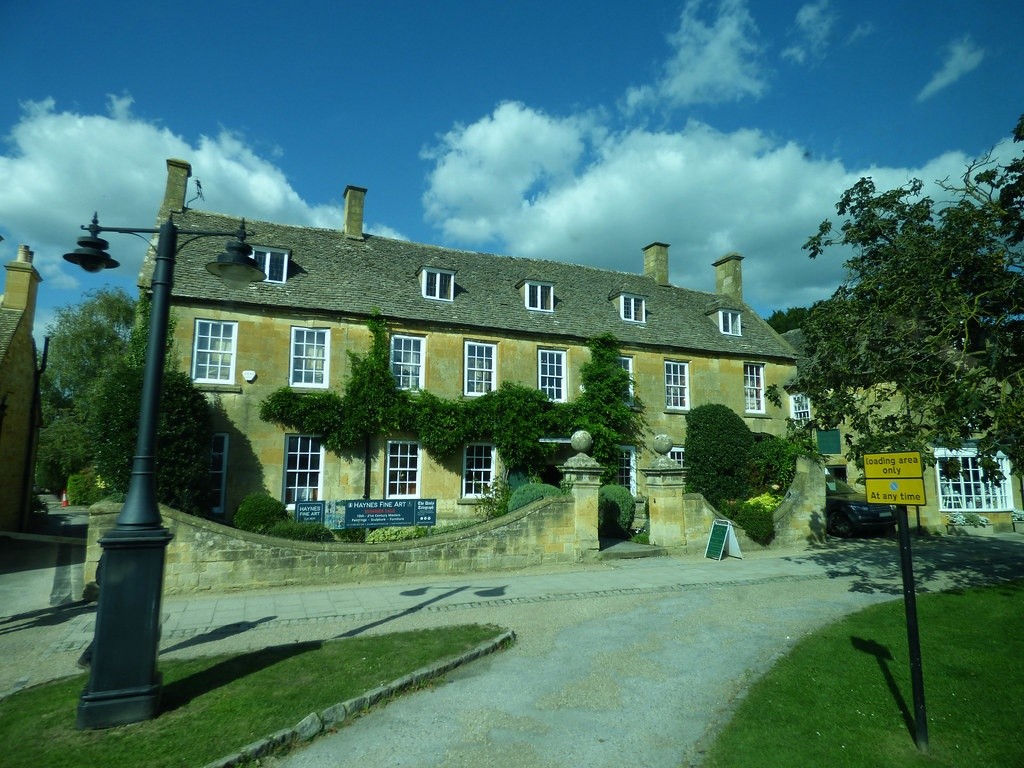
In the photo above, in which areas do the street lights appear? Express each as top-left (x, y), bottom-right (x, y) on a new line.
top-left (59, 210), bottom-right (268, 729)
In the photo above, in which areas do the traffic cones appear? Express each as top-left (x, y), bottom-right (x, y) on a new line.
top-left (61, 490), bottom-right (70, 507)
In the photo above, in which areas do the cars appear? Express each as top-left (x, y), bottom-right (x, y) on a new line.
top-left (825, 474), bottom-right (898, 540)
